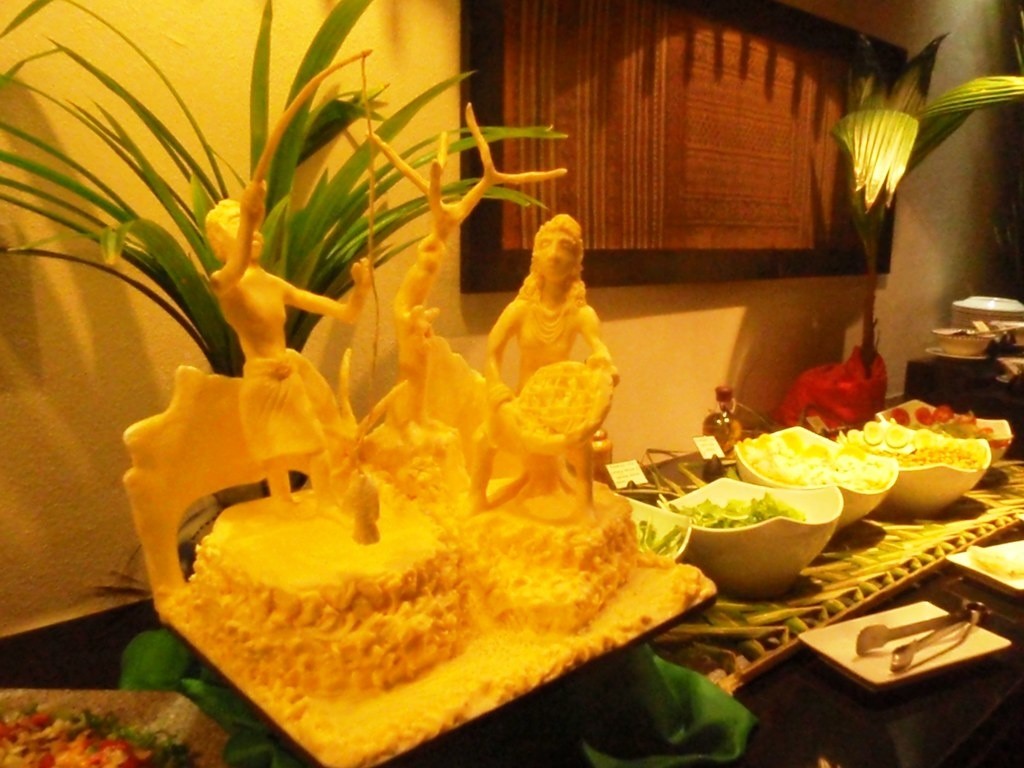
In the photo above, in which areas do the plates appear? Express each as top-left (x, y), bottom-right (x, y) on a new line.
top-left (797, 601), bottom-right (1011, 693)
top-left (943, 540), bottom-right (1024, 598)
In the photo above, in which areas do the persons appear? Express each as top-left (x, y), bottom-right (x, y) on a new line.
top-left (469, 213), bottom-right (618, 515)
top-left (205, 199), bottom-right (374, 514)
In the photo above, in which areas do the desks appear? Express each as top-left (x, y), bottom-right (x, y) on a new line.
top-left (0, 392), bottom-right (1024, 768)
top-left (903, 343), bottom-right (1024, 456)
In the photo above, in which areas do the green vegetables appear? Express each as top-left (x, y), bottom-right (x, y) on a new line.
top-left (669, 490), bottom-right (805, 529)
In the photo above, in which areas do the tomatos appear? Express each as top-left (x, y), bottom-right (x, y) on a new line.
top-left (890, 405), bottom-right (995, 435)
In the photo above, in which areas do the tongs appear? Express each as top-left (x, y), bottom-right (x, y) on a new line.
top-left (856, 601), bottom-right (987, 674)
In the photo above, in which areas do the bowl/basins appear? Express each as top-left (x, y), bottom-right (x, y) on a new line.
top-left (656, 476), bottom-right (844, 602)
top-left (875, 399), bottom-right (1013, 466)
top-left (934, 333), bottom-right (993, 356)
top-left (733, 424), bottom-right (899, 532)
top-left (835, 418), bottom-right (992, 520)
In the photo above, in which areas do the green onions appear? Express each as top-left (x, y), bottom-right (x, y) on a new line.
top-left (20, 704), bottom-right (197, 768)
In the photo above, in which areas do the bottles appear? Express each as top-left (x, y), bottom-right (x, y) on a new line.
top-left (703, 386), bottom-right (745, 484)
